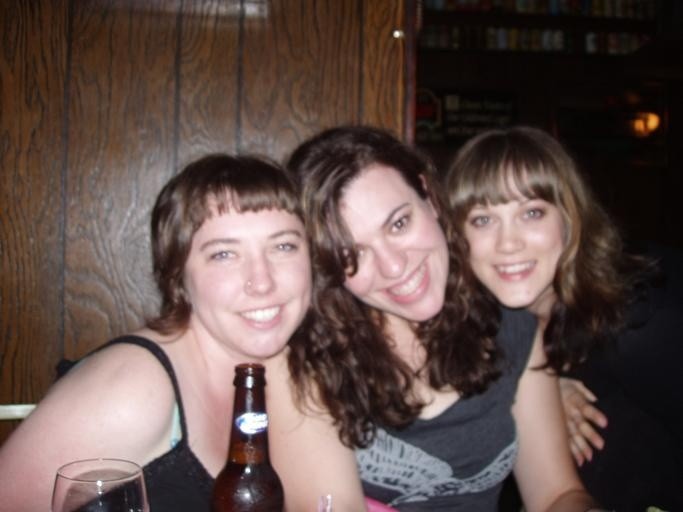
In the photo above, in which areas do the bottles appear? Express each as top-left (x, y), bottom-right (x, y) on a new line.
top-left (210, 360), bottom-right (285, 511)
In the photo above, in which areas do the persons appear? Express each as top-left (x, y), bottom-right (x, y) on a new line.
top-left (285, 120), bottom-right (600, 512)
top-left (449, 127), bottom-right (677, 512)
top-left (0, 151), bottom-right (368, 512)
